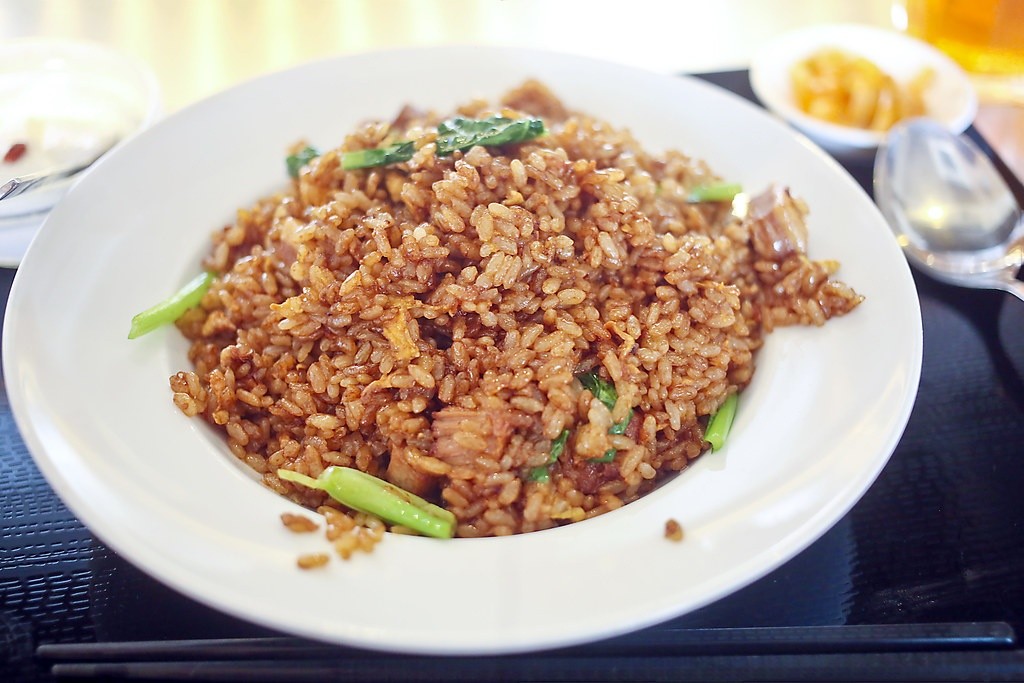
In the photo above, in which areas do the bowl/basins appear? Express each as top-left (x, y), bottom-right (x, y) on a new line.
top-left (746, 22), bottom-right (981, 180)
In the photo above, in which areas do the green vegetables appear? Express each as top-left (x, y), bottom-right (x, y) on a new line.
top-left (277, 464), bottom-right (457, 539)
top-left (128, 273), bottom-right (211, 339)
top-left (675, 182), bottom-right (741, 203)
top-left (579, 370), bottom-right (634, 459)
top-left (339, 115), bottom-right (545, 170)
top-left (702, 391), bottom-right (738, 453)
top-left (524, 429), bottom-right (569, 482)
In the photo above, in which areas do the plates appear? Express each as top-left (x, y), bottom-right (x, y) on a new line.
top-left (1, 30), bottom-right (157, 270)
top-left (1, 41), bottom-right (924, 657)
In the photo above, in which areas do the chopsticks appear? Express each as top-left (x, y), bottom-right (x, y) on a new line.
top-left (33, 621), bottom-right (1024, 683)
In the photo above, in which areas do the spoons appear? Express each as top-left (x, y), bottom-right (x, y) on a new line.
top-left (873, 114), bottom-right (1024, 303)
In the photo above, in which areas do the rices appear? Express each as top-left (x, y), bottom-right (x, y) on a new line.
top-left (167, 77), bottom-right (865, 570)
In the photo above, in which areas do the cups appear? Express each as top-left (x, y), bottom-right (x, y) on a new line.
top-left (881, 0), bottom-right (1024, 105)
